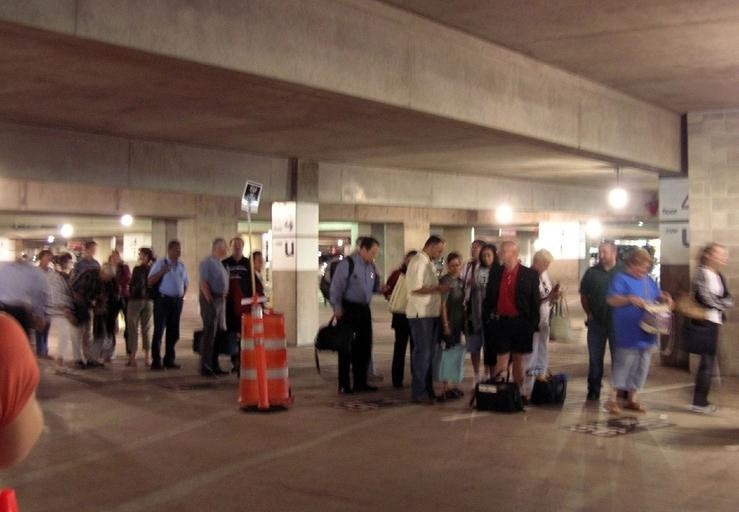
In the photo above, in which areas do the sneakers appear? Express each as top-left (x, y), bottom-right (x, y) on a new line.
top-left (586, 391), bottom-right (644, 414)
top-left (692, 405), bottom-right (716, 413)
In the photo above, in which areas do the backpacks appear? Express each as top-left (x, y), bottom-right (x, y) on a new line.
top-left (320, 256), bottom-right (354, 299)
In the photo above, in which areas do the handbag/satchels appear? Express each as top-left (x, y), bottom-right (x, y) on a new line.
top-left (194, 330), bottom-right (238, 355)
top-left (437, 345), bottom-right (467, 383)
top-left (476, 381), bottom-right (523, 413)
top-left (640, 303), bottom-right (672, 336)
top-left (316, 325), bottom-right (356, 354)
top-left (147, 285), bottom-right (159, 300)
top-left (674, 293), bottom-right (706, 321)
top-left (387, 275), bottom-right (408, 314)
top-left (531, 374), bottom-right (567, 405)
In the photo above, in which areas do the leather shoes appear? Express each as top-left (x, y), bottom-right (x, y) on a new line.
top-left (337, 376), bottom-right (464, 405)
top-left (127, 361), bottom-right (240, 379)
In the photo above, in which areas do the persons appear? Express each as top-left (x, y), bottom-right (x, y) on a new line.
top-left (601, 246), bottom-right (674, 416)
top-left (384, 235), bottom-right (563, 403)
top-left (680, 242), bottom-right (736, 416)
top-left (330, 236), bottom-right (383, 394)
top-left (579, 239), bottom-right (626, 402)
top-left (1, 238), bottom-right (268, 380)
top-left (1, 309), bottom-right (44, 472)
